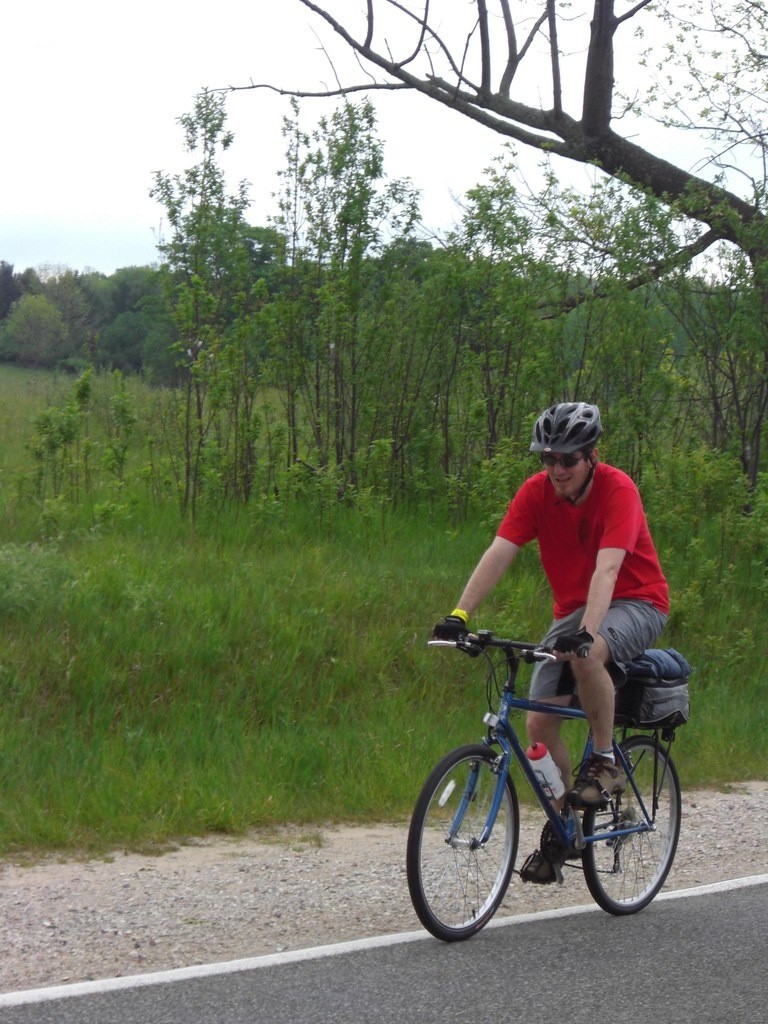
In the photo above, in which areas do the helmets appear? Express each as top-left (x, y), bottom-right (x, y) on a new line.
top-left (529, 401), bottom-right (601, 451)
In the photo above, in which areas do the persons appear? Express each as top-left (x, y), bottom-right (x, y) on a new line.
top-left (431, 402), bottom-right (671, 885)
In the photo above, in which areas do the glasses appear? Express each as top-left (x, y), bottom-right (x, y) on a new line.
top-left (540, 452), bottom-right (587, 468)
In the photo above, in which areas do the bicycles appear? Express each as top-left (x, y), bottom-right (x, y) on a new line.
top-left (405, 628), bottom-right (682, 943)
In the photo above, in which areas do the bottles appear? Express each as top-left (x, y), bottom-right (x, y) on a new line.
top-left (526, 742), bottom-right (566, 799)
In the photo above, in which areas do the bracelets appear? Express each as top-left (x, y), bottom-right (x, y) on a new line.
top-left (450, 608), bottom-right (468, 623)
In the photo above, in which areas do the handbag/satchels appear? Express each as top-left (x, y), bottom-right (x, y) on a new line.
top-left (616, 646), bottom-right (691, 726)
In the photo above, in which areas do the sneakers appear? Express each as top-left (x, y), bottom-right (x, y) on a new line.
top-left (521, 821), bottom-right (582, 883)
top-left (564, 752), bottom-right (626, 810)
